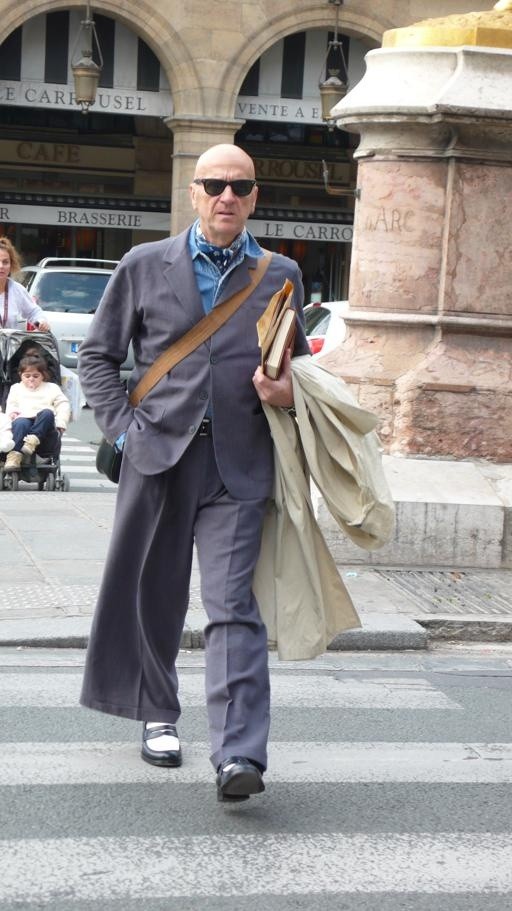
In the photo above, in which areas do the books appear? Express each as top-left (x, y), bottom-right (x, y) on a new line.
top-left (265, 307), bottom-right (311, 380)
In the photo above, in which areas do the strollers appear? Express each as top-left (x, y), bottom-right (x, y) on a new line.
top-left (1, 321), bottom-right (69, 495)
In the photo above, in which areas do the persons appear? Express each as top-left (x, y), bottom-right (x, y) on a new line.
top-left (0, 236), bottom-right (50, 412)
top-left (74, 141), bottom-right (312, 802)
top-left (4, 353), bottom-right (70, 469)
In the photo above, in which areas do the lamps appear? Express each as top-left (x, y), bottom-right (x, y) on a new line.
top-left (69, 1), bottom-right (106, 116)
top-left (317, 1), bottom-right (351, 133)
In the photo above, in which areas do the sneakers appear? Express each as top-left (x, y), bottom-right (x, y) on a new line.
top-left (4, 452), bottom-right (24, 470)
top-left (20, 434), bottom-right (41, 456)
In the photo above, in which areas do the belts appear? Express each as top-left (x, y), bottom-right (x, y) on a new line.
top-left (197, 415), bottom-right (218, 438)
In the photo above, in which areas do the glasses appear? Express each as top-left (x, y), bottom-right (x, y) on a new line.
top-left (193, 177), bottom-right (258, 196)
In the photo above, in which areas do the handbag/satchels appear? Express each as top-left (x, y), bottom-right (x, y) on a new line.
top-left (96, 398), bottom-right (124, 483)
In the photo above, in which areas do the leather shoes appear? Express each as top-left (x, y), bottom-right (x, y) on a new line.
top-left (216, 760), bottom-right (266, 803)
top-left (141, 719), bottom-right (185, 767)
